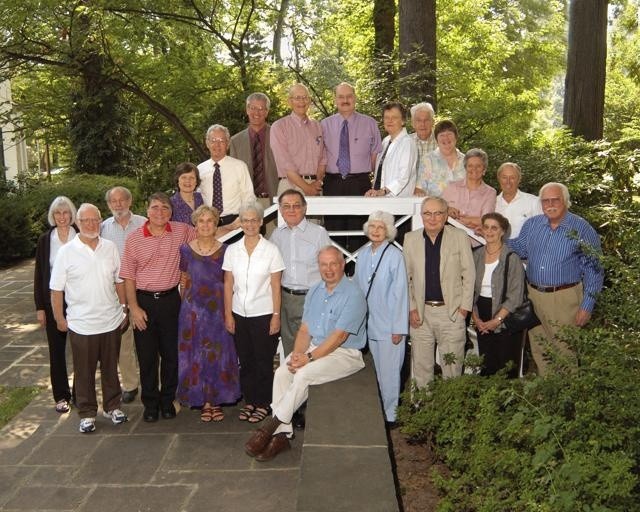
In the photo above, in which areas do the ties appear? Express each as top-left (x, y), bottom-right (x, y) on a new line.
top-left (254, 133), bottom-right (264, 194)
top-left (213, 163), bottom-right (223, 215)
top-left (337, 120), bottom-right (350, 178)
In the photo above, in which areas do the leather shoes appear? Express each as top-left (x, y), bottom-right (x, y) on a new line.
top-left (161, 405), bottom-right (176, 418)
top-left (293, 411), bottom-right (304, 431)
top-left (143, 408), bottom-right (159, 423)
top-left (246, 416), bottom-right (289, 462)
top-left (123, 388), bottom-right (138, 403)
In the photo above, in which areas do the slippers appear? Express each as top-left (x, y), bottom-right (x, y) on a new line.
top-left (56, 400), bottom-right (69, 412)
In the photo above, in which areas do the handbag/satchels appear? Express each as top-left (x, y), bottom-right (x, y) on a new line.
top-left (503, 299), bottom-right (541, 333)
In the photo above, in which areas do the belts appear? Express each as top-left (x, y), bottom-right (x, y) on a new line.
top-left (219, 218), bottom-right (224, 225)
top-left (140, 290), bottom-right (176, 298)
top-left (281, 286), bottom-right (308, 295)
top-left (335, 173), bottom-right (359, 179)
top-left (528, 281), bottom-right (580, 292)
top-left (257, 193), bottom-right (269, 198)
top-left (279, 175), bottom-right (317, 179)
top-left (425, 302), bottom-right (444, 305)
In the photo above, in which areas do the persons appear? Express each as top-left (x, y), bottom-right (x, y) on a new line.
top-left (34, 83), bottom-right (606, 462)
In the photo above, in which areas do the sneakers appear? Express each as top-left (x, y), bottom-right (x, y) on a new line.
top-left (103, 408), bottom-right (127, 424)
top-left (80, 417), bottom-right (95, 433)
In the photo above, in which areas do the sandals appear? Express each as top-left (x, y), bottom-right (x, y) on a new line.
top-left (239, 403), bottom-right (271, 423)
top-left (201, 408), bottom-right (224, 422)
